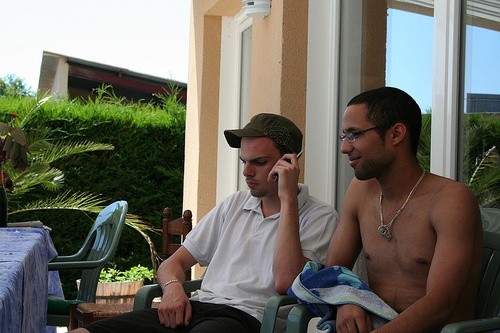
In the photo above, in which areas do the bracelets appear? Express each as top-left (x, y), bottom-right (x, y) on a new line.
top-left (162, 280), bottom-right (183, 290)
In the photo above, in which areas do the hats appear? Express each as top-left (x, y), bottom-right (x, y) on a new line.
top-left (223, 112), bottom-right (303, 159)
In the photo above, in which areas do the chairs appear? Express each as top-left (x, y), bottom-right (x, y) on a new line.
top-left (68, 207), bottom-right (192, 333)
top-left (45, 199), bottom-right (128, 330)
top-left (285, 207), bottom-right (500, 333)
top-left (132, 263), bottom-right (300, 333)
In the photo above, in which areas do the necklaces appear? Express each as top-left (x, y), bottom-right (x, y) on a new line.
top-left (377, 169), bottom-right (426, 240)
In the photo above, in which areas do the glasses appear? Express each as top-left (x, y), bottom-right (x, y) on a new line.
top-left (338, 119), bottom-right (399, 143)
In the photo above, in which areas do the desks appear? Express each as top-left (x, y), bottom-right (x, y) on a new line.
top-left (0, 223), bottom-right (64, 333)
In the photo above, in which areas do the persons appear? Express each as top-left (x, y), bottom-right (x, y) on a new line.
top-left (65, 114), bottom-right (338, 333)
top-left (325, 87), bottom-right (484, 333)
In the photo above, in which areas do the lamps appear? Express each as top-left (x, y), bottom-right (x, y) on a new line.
top-left (242, 0), bottom-right (272, 19)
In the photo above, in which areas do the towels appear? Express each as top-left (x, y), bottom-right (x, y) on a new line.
top-left (287, 260), bottom-right (401, 333)
